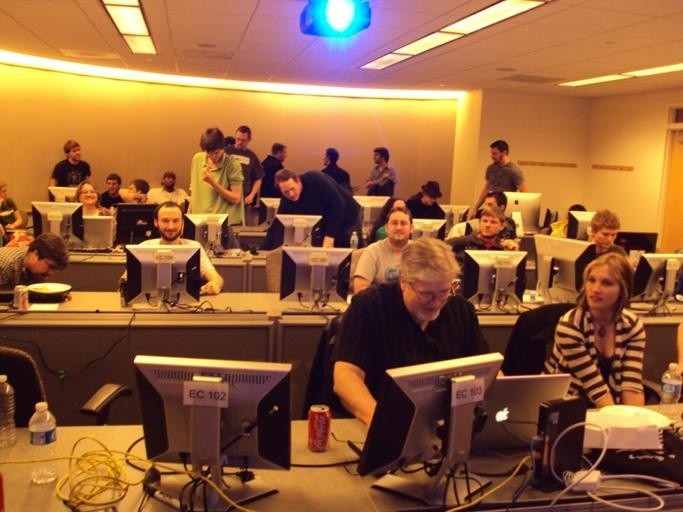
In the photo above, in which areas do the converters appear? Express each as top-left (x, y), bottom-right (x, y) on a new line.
top-left (572, 470), bottom-right (602, 491)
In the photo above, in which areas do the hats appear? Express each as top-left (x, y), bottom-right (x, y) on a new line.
top-left (421, 181), bottom-right (442, 198)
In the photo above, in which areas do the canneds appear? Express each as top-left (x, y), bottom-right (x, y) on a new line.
top-left (13, 285), bottom-right (29, 314)
top-left (309, 405), bottom-right (331, 453)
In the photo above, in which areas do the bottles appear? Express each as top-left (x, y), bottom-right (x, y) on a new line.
top-left (0, 374), bottom-right (16, 451)
top-left (349, 231), bottom-right (358, 248)
top-left (657, 362), bottom-right (683, 421)
top-left (27, 401), bottom-right (58, 485)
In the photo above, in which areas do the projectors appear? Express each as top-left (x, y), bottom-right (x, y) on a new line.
top-left (299, 0), bottom-right (371, 40)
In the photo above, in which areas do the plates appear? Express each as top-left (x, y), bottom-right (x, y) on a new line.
top-left (25, 280), bottom-right (72, 295)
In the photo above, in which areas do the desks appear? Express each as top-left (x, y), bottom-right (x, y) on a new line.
top-left (1, 223), bottom-right (682, 511)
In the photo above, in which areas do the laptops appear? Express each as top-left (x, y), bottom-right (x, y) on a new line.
top-left (470, 374), bottom-right (573, 455)
top-left (0, 283), bottom-right (71, 304)
top-left (81, 215), bottom-right (119, 253)
top-left (615, 232), bottom-right (658, 255)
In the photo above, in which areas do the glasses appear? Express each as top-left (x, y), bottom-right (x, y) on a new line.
top-left (409, 282), bottom-right (456, 303)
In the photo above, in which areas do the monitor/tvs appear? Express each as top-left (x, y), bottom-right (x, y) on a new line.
top-left (352, 194), bottom-right (392, 240)
top-left (631, 253), bottom-right (683, 316)
top-left (461, 249), bottom-right (528, 315)
top-left (184, 213), bottom-right (230, 255)
top-left (534, 234), bottom-right (596, 306)
top-left (438, 204), bottom-right (472, 237)
top-left (115, 202), bottom-right (162, 245)
top-left (31, 201), bottom-right (85, 251)
top-left (270, 214), bottom-right (324, 246)
top-left (566, 211), bottom-right (602, 242)
top-left (409, 219), bottom-right (447, 243)
top-left (503, 191), bottom-right (541, 237)
top-left (279, 247), bottom-right (352, 313)
top-left (257, 198), bottom-right (284, 231)
top-left (49, 186), bottom-right (80, 203)
top-left (133, 355), bottom-right (292, 512)
top-left (125, 245), bottom-right (200, 309)
top-left (357, 352), bottom-right (492, 509)
